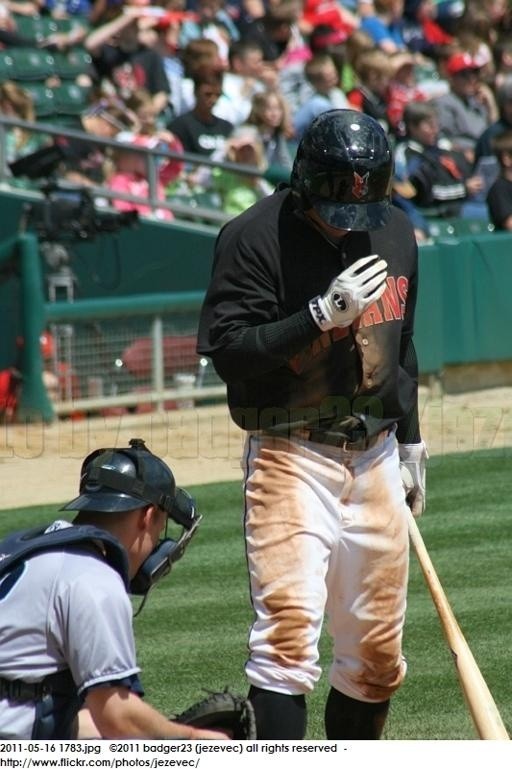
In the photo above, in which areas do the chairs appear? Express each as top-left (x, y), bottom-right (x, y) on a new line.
top-left (1, 0), bottom-right (110, 206)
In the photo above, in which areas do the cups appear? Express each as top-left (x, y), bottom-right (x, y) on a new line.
top-left (173, 373), bottom-right (195, 409)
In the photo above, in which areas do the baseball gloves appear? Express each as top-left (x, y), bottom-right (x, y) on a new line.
top-left (171, 691), bottom-right (257, 740)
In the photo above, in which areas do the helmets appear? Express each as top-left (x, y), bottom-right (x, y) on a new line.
top-left (291, 108), bottom-right (394, 231)
top-left (59, 439), bottom-right (202, 594)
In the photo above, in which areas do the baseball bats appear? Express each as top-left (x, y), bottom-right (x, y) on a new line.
top-left (403, 503), bottom-right (510, 740)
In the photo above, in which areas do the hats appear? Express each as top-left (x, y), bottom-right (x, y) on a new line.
top-left (447, 53), bottom-right (483, 75)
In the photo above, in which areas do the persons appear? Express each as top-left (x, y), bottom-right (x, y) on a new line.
top-left (2, 2), bottom-right (511, 245)
top-left (0, 433), bottom-right (260, 739)
top-left (188, 102), bottom-right (433, 742)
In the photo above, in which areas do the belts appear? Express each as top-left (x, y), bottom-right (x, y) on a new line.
top-left (289, 428), bottom-right (390, 451)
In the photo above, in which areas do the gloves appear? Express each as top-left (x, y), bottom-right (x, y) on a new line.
top-left (399, 441), bottom-right (429, 517)
top-left (308, 255), bottom-right (388, 332)
